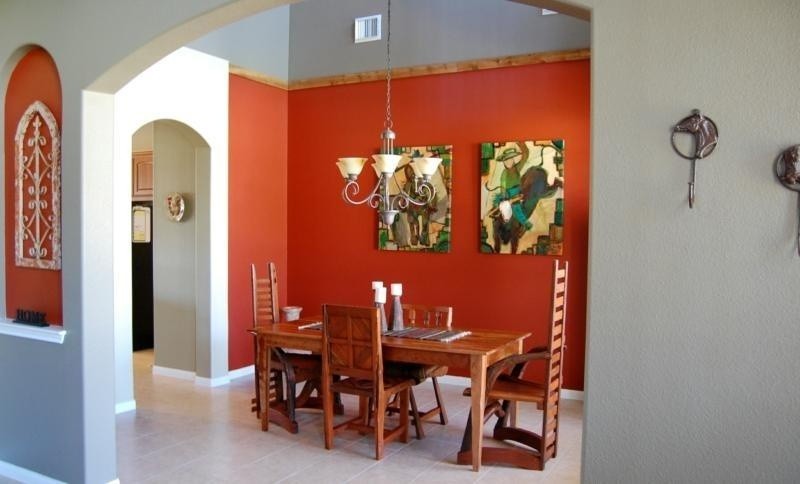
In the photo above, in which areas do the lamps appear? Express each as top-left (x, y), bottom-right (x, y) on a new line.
top-left (336, 0), bottom-right (442, 231)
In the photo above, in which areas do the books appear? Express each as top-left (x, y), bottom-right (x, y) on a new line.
top-left (382, 326), bottom-right (471, 342)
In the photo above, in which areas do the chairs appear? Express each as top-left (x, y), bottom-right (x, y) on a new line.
top-left (250, 261), bottom-right (345, 435)
top-left (458, 259), bottom-right (568, 470)
top-left (322, 303), bottom-right (411, 460)
top-left (366, 305), bottom-right (451, 439)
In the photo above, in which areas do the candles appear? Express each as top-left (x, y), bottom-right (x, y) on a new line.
top-left (391, 284), bottom-right (402, 296)
top-left (375, 288), bottom-right (386, 303)
top-left (372, 281), bottom-right (384, 289)
